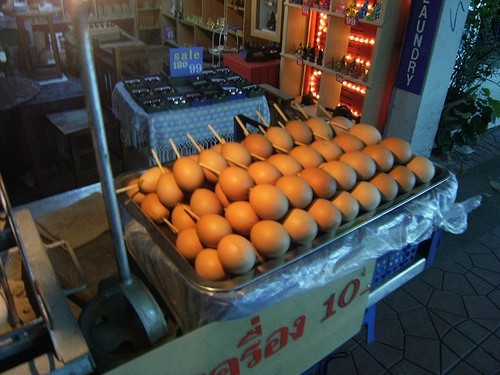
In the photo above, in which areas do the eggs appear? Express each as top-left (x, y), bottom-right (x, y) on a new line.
top-left (127, 116), bottom-right (435, 282)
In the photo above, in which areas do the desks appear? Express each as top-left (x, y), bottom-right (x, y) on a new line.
top-left (111, 67), bottom-right (270, 166)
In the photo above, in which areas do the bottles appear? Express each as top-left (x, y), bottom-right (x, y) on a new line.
top-left (336, 54), bottom-right (371, 83)
top-left (266, 11), bottom-right (276, 31)
top-left (296, 42), bottom-right (323, 66)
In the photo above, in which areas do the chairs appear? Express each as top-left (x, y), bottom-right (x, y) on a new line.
top-left (0, 12), bottom-right (123, 187)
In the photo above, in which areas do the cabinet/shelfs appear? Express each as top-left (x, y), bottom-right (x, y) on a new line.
top-left (279, 1), bottom-right (401, 127)
top-left (51, 0), bottom-right (257, 63)
